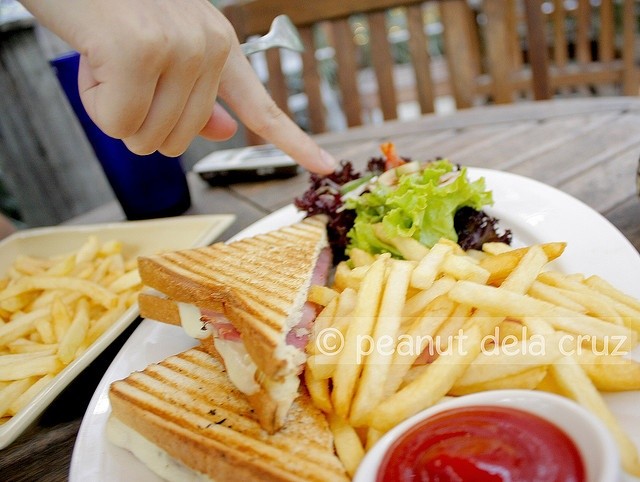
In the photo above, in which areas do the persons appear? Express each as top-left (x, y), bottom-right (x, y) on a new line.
top-left (20, 2), bottom-right (338, 178)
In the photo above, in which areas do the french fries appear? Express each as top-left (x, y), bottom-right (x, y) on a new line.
top-left (308, 215), bottom-right (640, 482)
top-left (0, 234), bottom-right (144, 425)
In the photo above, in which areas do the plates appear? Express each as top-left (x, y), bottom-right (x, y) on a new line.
top-left (0, 215), bottom-right (237, 451)
top-left (68, 168), bottom-right (640, 482)
top-left (354, 389), bottom-right (618, 482)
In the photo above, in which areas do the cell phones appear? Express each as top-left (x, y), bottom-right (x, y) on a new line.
top-left (194, 144), bottom-right (297, 186)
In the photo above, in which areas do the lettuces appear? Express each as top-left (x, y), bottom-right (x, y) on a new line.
top-left (336, 158), bottom-right (493, 264)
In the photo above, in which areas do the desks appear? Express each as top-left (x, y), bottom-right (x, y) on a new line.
top-left (0, 99), bottom-right (639, 481)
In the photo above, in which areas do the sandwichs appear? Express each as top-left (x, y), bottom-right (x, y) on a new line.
top-left (104, 343), bottom-right (360, 482)
top-left (133, 213), bottom-right (350, 431)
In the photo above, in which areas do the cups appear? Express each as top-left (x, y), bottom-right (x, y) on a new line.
top-left (49, 50), bottom-right (191, 221)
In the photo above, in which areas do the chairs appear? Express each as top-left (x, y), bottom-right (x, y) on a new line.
top-left (221, 1), bottom-right (482, 148)
top-left (474, 1), bottom-right (640, 105)
